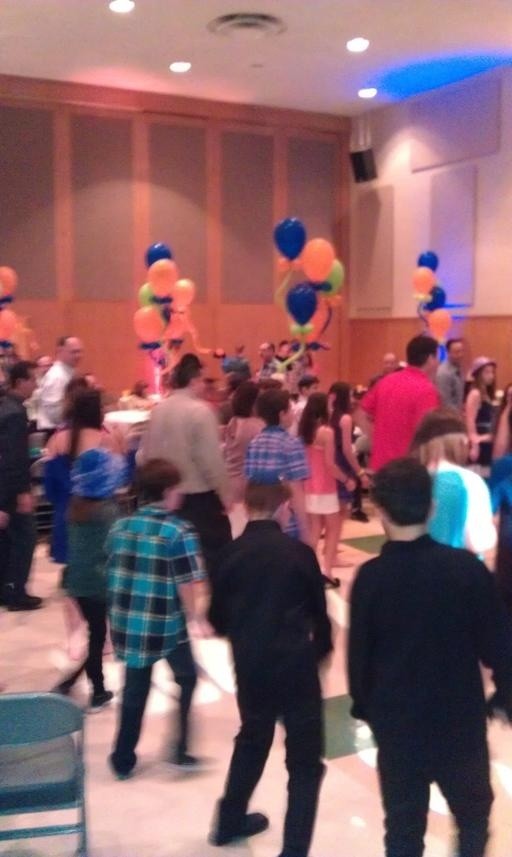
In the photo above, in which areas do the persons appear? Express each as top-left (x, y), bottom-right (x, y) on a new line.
top-left (207, 468), bottom-right (335, 857)
top-left (99, 457), bottom-right (206, 782)
top-left (1, 329), bottom-right (511, 726)
top-left (347, 458), bottom-right (511, 856)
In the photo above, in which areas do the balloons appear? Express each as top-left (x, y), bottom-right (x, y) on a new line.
top-left (1, 264), bottom-right (20, 349)
top-left (131, 241), bottom-right (196, 369)
top-left (409, 250), bottom-right (453, 365)
top-left (271, 215), bottom-right (345, 353)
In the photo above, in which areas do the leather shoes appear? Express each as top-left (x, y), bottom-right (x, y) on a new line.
top-left (207, 813), bottom-right (268, 845)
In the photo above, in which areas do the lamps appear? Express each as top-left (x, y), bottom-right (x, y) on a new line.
top-left (208, 10), bottom-right (286, 97)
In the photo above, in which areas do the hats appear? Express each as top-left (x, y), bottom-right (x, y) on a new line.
top-left (72, 451), bottom-right (118, 499)
top-left (471, 356), bottom-right (496, 374)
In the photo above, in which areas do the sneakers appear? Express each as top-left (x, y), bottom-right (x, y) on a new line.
top-left (90, 691), bottom-right (113, 709)
top-left (8, 596), bottom-right (42, 611)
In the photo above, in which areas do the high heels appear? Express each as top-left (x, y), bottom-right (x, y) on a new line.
top-left (322, 574), bottom-right (340, 587)
